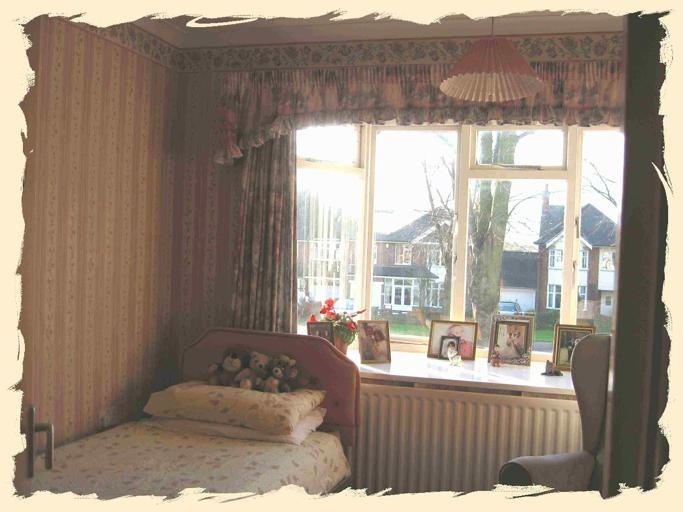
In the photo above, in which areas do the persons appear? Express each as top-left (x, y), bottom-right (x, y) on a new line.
top-left (566, 338), bottom-right (580, 362)
top-left (444, 341), bottom-right (455, 358)
top-left (315, 328), bottom-right (331, 341)
top-left (499, 331), bottom-right (524, 358)
top-left (362, 326), bottom-right (387, 360)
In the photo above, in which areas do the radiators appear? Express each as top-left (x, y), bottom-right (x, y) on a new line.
top-left (350, 383), bottom-right (582, 494)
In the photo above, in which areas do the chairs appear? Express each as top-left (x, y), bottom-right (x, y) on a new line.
top-left (498, 332), bottom-right (615, 493)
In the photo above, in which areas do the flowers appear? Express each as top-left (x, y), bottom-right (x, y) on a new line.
top-left (309, 297), bottom-right (366, 343)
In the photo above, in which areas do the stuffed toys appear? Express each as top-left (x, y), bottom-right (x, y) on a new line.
top-left (206, 344), bottom-right (297, 393)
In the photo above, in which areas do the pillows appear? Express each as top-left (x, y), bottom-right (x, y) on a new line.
top-left (142, 378), bottom-right (327, 449)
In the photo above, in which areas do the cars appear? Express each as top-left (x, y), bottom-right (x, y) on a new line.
top-left (499, 301), bottom-right (523, 315)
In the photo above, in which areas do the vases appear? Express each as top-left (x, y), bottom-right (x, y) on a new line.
top-left (333, 324), bottom-right (348, 354)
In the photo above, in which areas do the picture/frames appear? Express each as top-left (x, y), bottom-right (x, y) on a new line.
top-left (308, 321), bottom-right (333, 343)
top-left (552, 325), bottom-right (597, 370)
top-left (488, 315), bottom-right (534, 365)
top-left (426, 321), bottom-right (478, 361)
top-left (357, 318), bottom-right (390, 364)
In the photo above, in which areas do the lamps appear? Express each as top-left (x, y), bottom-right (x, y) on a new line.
top-left (442, 16), bottom-right (541, 104)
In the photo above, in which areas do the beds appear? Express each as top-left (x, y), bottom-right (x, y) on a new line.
top-left (18, 329), bottom-right (360, 497)
